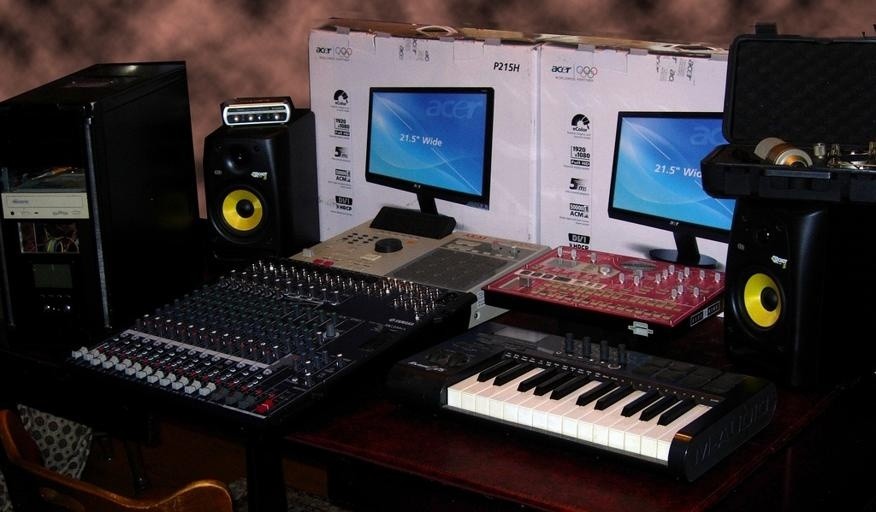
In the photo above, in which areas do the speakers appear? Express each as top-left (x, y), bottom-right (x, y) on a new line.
top-left (724, 197), bottom-right (876, 391)
top-left (204, 109), bottom-right (318, 280)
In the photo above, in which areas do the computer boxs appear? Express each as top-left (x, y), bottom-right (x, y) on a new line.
top-left (0, 60), bottom-right (202, 340)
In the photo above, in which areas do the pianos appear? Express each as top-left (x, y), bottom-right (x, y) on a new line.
top-left (383, 320), bottom-right (777, 491)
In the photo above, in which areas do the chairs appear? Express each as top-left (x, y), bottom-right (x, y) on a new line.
top-left (1, 407), bottom-right (235, 511)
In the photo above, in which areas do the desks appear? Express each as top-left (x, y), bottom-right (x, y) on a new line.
top-left (1, 271), bottom-right (874, 511)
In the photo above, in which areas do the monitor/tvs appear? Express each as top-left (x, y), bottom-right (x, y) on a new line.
top-left (608, 112), bottom-right (736, 269)
top-left (365, 87), bottom-right (494, 241)
